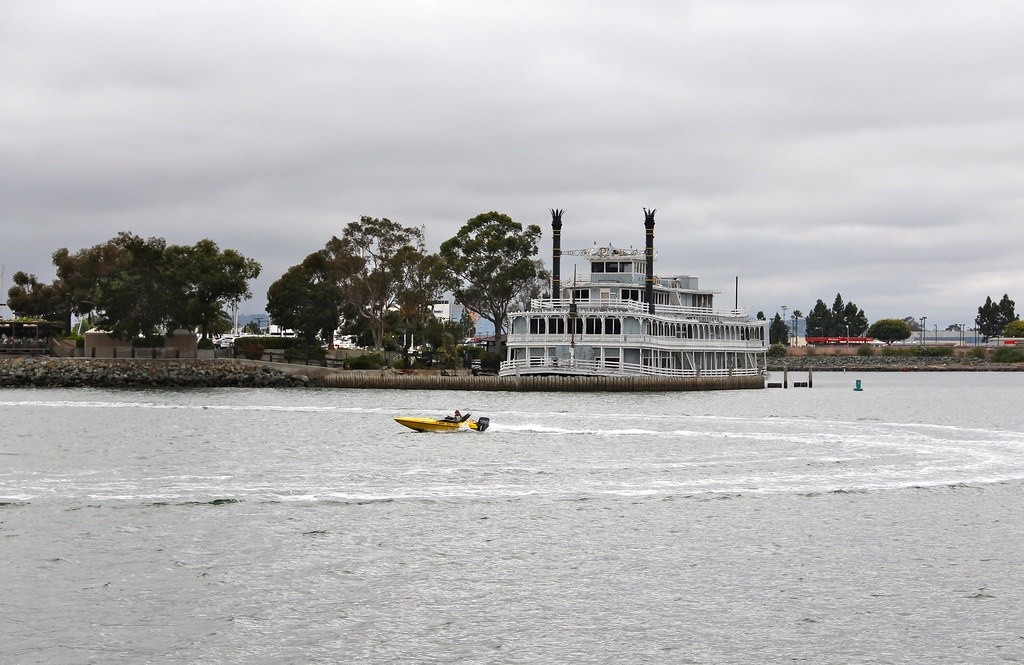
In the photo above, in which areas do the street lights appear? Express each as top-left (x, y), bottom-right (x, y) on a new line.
top-left (781, 305), bottom-right (789, 321)
top-left (934, 323), bottom-right (938, 342)
top-left (959, 323), bottom-right (966, 346)
top-left (919, 317), bottom-right (924, 344)
top-left (923, 316), bottom-right (927, 345)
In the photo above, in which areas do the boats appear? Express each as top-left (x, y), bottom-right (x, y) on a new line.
top-left (394, 410), bottom-right (490, 433)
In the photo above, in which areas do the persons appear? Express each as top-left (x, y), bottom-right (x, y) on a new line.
top-left (381, 366), bottom-right (388, 383)
top-left (454, 410), bottom-right (462, 422)
